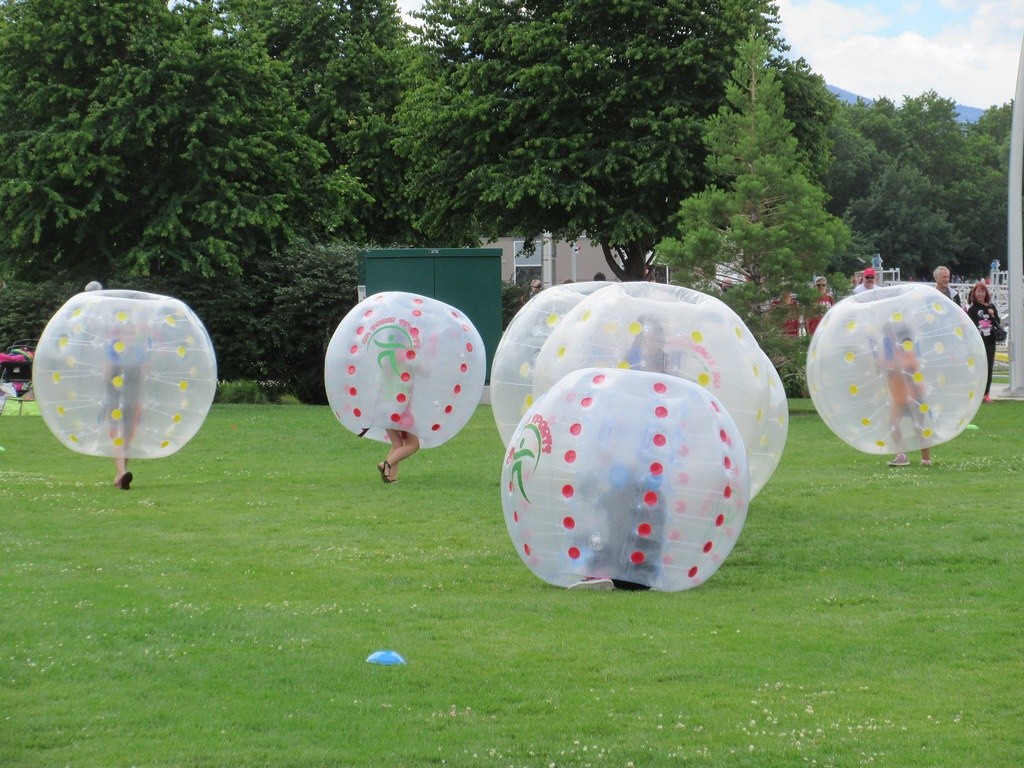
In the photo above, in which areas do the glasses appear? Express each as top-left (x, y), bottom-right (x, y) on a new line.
top-left (864, 277), bottom-right (875, 280)
top-left (816, 283), bottom-right (826, 288)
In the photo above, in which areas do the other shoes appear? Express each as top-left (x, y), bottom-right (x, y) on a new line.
top-left (114, 472), bottom-right (132, 490)
top-left (983, 394), bottom-right (994, 404)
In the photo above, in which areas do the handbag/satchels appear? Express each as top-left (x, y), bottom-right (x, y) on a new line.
top-left (988, 303), bottom-right (1007, 342)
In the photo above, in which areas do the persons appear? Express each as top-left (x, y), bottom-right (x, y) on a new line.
top-left (520, 272), bottom-right (605, 304)
top-left (868, 321), bottom-right (933, 471)
top-left (96, 313), bottom-right (150, 488)
top-left (565, 456), bottom-right (668, 592)
top-left (619, 313), bottom-right (693, 477)
top-left (376, 325), bottom-right (441, 483)
top-left (770, 266), bottom-right (1005, 404)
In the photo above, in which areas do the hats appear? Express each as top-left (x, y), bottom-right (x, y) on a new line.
top-left (864, 268), bottom-right (876, 277)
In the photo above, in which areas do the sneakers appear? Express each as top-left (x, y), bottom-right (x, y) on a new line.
top-left (568, 575), bottom-right (613, 591)
top-left (886, 454), bottom-right (909, 465)
top-left (921, 459), bottom-right (931, 465)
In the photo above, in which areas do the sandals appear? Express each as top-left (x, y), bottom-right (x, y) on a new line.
top-left (377, 461), bottom-right (397, 483)
